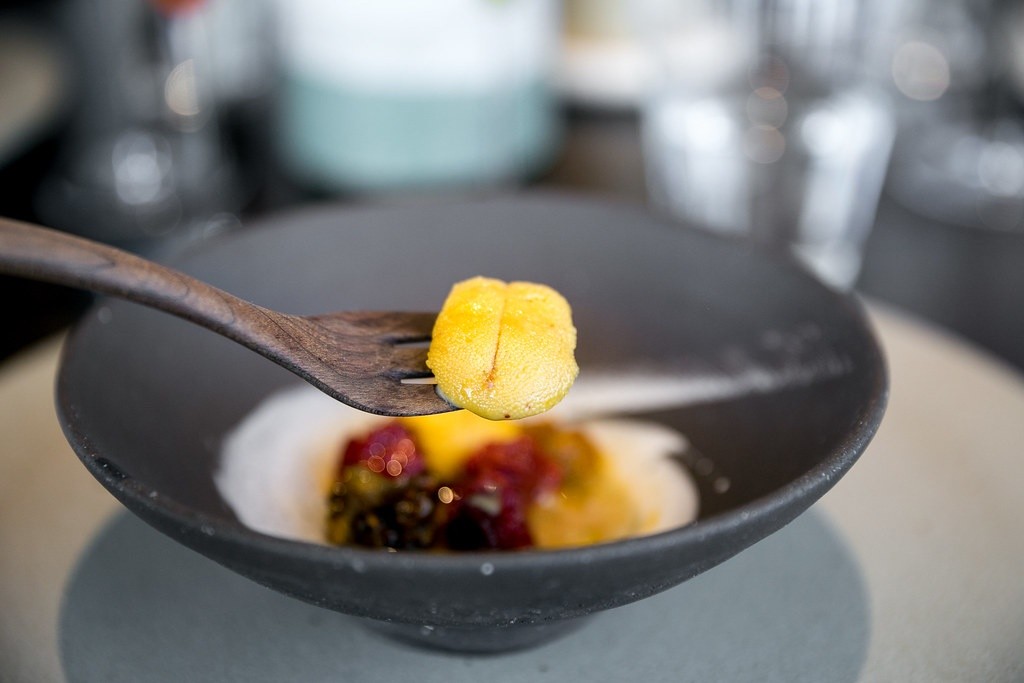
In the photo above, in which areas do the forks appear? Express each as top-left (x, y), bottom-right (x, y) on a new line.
top-left (0, 213), bottom-right (465, 416)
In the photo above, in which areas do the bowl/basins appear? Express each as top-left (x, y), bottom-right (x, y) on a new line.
top-left (55, 191), bottom-right (889, 652)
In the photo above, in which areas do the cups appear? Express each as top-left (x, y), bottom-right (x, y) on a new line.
top-left (640, 0), bottom-right (918, 288)
